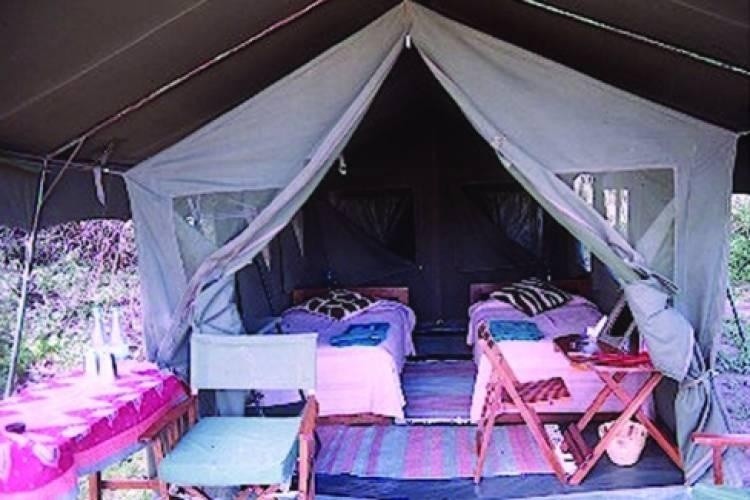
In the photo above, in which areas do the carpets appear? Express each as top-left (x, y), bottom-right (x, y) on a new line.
top-left (311, 354), bottom-right (584, 480)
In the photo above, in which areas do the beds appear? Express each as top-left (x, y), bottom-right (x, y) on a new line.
top-left (466, 276), bottom-right (660, 456)
top-left (245, 285), bottom-right (415, 425)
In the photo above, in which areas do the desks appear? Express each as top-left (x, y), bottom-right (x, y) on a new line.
top-left (552, 334), bottom-right (689, 486)
top-left (1, 358), bottom-right (186, 500)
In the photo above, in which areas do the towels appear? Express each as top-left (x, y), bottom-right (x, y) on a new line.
top-left (330, 323), bottom-right (388, 346)
top-left (489, 317), bottom-right (543, 341)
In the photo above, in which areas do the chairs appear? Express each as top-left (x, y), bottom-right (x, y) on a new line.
top-left (470, 319), bottom-right (575, 484)
top-left (692, 434), bottom-right (746, 500)
top-left (138, 332), bottom-right (324, 500)
